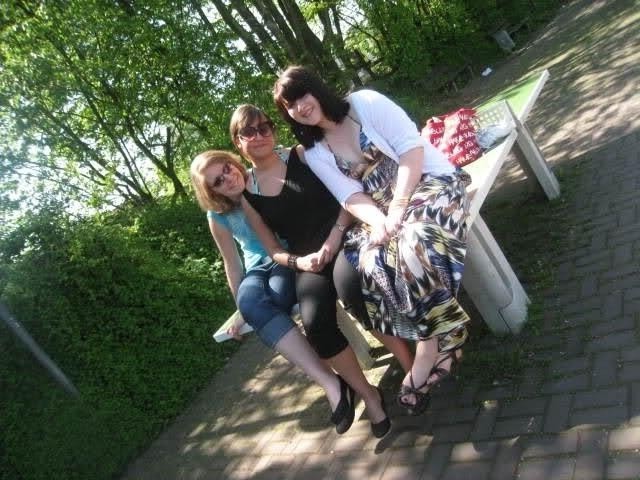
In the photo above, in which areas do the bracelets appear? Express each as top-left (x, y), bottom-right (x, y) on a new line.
top-left (391, 196), bottom-right (411, 207)
top-left (288, 256), bottom-right (298, 272)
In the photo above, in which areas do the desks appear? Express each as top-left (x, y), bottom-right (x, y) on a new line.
top-left (213, 70), bottom-right (558, 344)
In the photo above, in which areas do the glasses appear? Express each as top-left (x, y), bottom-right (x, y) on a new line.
top-left (237, 121), bottom-right (276, 143)
top-left (210, 162), bottom-right (232, 189)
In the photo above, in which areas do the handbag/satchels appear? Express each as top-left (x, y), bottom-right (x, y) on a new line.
top-left (423, 107), bottom-right (484, 168)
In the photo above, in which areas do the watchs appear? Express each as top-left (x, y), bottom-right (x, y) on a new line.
top-left (334, 221), bottom-right (347, 234)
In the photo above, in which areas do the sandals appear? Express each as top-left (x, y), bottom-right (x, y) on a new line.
top-left (395, 367), bottom-right (433, 410)
top-left (427, 349), bottom-right (459, 388)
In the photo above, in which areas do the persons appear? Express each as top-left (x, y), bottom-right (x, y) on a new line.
top-left (229, 102), bottom-right (415, 438)
top-left (272, 64), bottom-right (473, 417)
top-left (190, 149), bottom-right (355, 433)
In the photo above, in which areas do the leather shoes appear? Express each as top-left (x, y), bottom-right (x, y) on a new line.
top-left (330, 373), bottom-right (351, 425)
top-left (336, 385), bottom-right (356, 434)
top-left (371, 387), bottom-right (392, 438)
